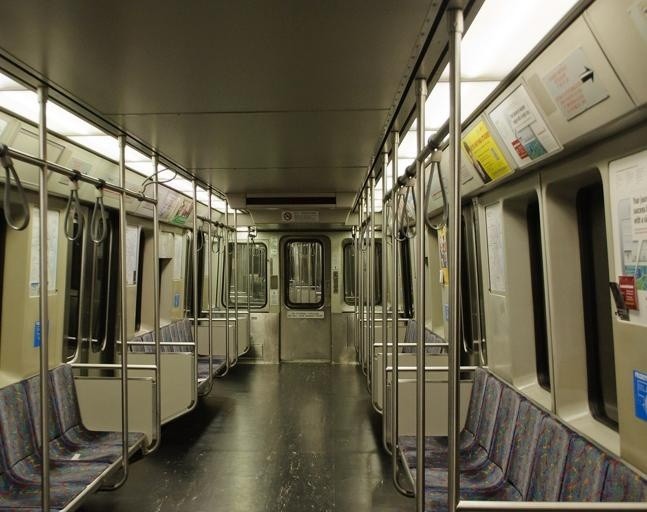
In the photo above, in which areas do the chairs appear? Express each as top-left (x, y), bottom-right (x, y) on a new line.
top-left (0, 364), bottom-right (147, 512)
top-left (398, 367), bottom-right (647, 512)
top-left (130, 319), bottom-right (226, 391)
top-left (401, 320), bottom-right (448, 353)
